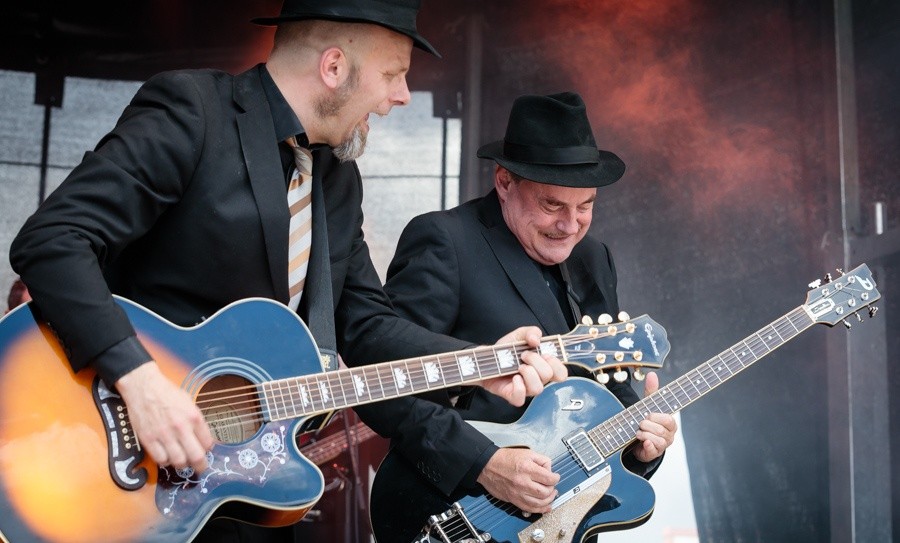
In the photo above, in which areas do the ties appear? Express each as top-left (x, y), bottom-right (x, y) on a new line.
top-left (281, 134), bottom-right (319, 314)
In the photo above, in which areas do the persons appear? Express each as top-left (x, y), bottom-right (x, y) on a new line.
top-left (351, 92), bottom-right (676, 543)
top-left (5, 274), bottom-right (35, 319)
top-left (0, 0), bottom-right (568, 543)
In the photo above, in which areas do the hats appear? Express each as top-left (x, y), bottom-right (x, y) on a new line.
top-left (247, 0), bottom-right (443, 60)
top-left (476, 90), bottom-right (626, 189)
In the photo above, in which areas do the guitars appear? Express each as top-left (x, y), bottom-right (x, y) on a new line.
top-left (370, 261), bottom-right (882, 543)
top-left (0, 295), bottom-right (670, 542)
